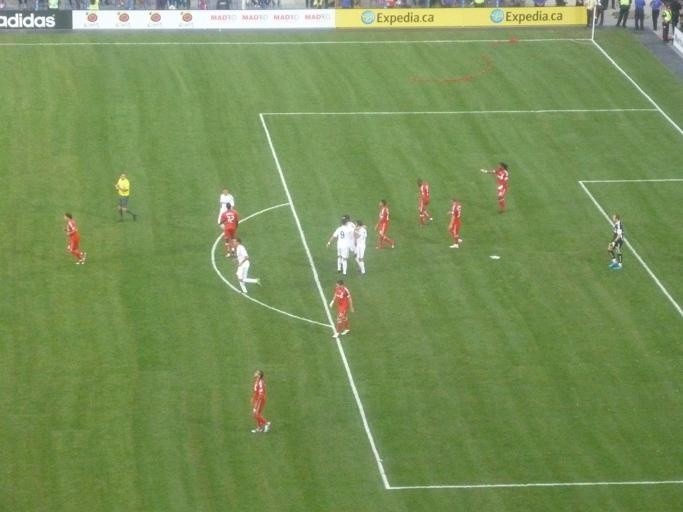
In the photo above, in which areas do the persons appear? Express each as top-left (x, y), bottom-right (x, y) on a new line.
top-left (217, 188), bottom-right (235, 231)
top-left (217, 205), bottom-right (239, 259)
top-left (352, 220), bottom-right (366, 277)
top-left (328, 282), bottom-right (355, 337)
top-left (415, 178), bottom-right (432, 226)
top-left (325, 218), bottom-right (355, 278)
top-left (112, 173), bottom-right (137, 223)
top-left (249, 370), bottom-right (271, 435)
top-left (341, 214), bottom-right (356, 256)
top-left (444, 197), bottom-right (463, 249)
top-left (584, 0), bottom-right (682, 43)
top-left (373, 199), bottom-right (395, 251)
top-left (480, 162), bottom-right (508, 214)
top-left (63, 213), bottom-right (86, 265)
top-left (234, 238), bottom-right (261, 294)
top-left (0, 0), bottom-right (584, 10)
top-left (607, 214), bottom-right (624, 269)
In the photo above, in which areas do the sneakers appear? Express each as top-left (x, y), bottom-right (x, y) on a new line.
top-left (251, 421), bottom-right (271, 433)
top-left (242, 278), bottom-right (262, 294)
top-left (338, 240), bottom-right (396, 277)
top-left (76, 251), bottom-right (87, 265)
top-left (117, 215), bottom-right (136, 222)
top-left (609, 262), bottom-right (622, 270)
top-left (225, 252), bottom-right (236, 258)
top-left (420, 217), bottom-right (462, 249)
top-left (331, 330), bottom-right (350, 338)
